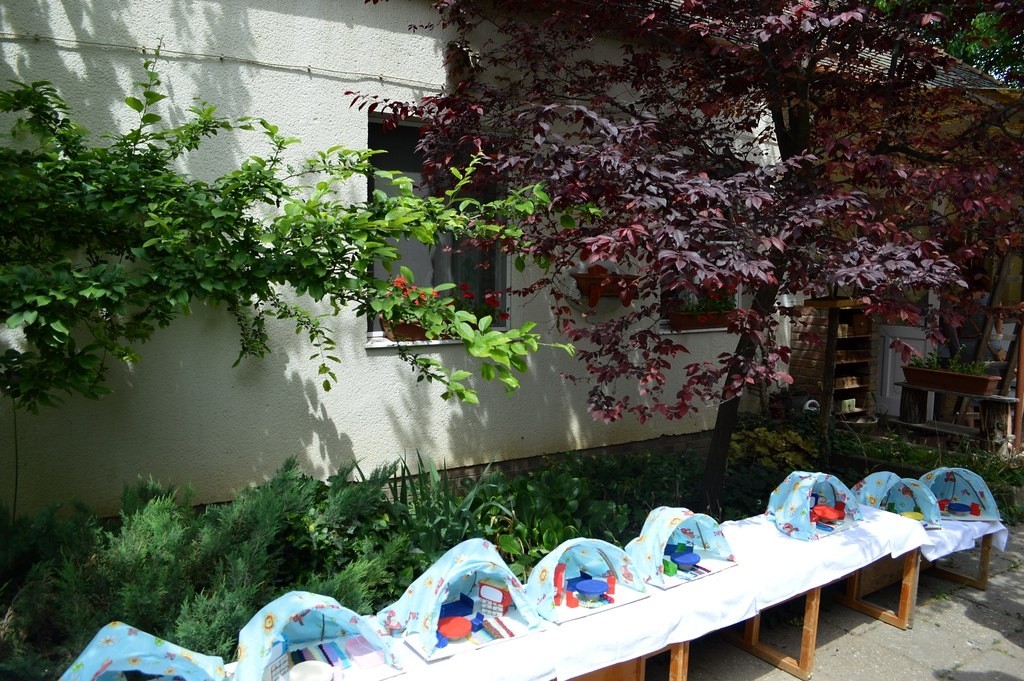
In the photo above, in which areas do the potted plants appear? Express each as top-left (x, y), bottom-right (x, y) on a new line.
top-left (899, 354), bottom-right (1001, 394)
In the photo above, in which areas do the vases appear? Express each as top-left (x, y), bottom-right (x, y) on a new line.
top-left (670, 310), bottom-right (736, 329)
top-left (377, 313), bottom-right (458, 342)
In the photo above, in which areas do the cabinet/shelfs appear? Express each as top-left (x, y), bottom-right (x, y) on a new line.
top-left (787, 299), bottom-right (881, 433)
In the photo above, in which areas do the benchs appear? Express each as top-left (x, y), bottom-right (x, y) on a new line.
top-left (893, 381), bottom-right (1020, 439)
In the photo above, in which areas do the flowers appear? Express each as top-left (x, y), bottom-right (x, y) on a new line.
top-left (385, 271), bottom-right (446, 314)
top-left (677, 281), bottom-right (735, 322)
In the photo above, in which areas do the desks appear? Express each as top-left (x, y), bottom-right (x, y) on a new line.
top-left (218, 505), bottom-right (1009, 681)
